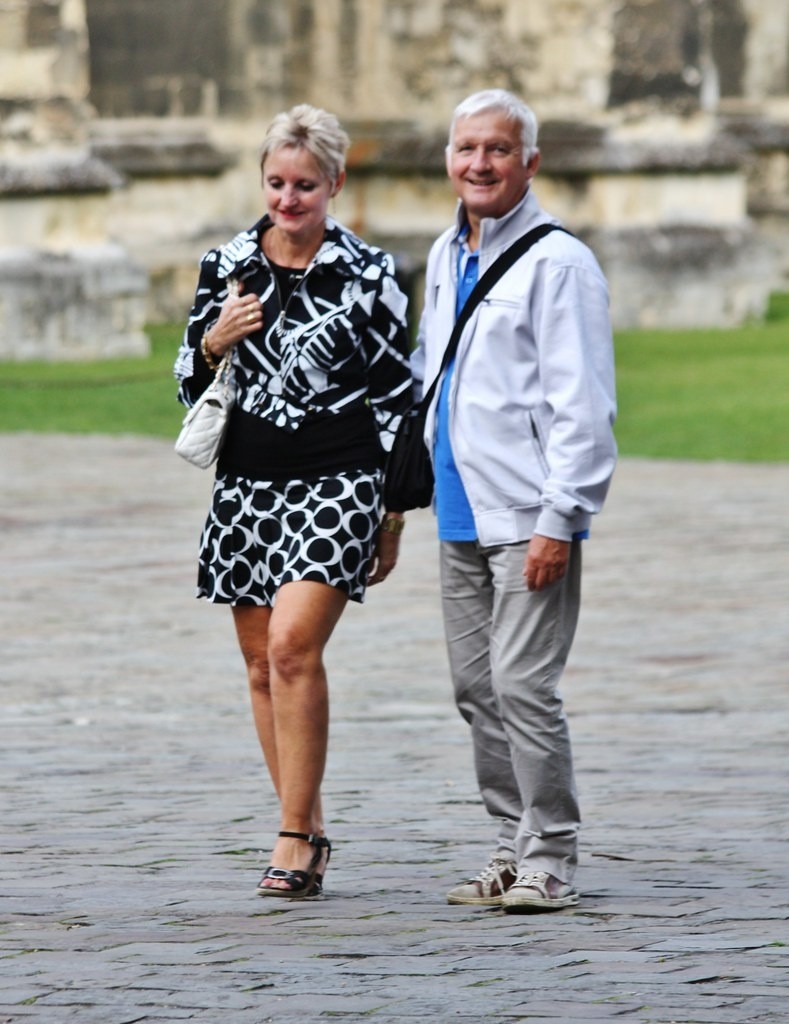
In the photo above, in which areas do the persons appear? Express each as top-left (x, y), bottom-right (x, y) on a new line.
top-left (173, 101), bottom-right (411, 900)
top-left (410, 93), bottom-right (617, 915)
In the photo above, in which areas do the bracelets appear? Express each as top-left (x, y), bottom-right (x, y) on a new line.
top-left (380, 516), bottom-right (405, 535)
top-left (199, 334), bottom-right (220, 374)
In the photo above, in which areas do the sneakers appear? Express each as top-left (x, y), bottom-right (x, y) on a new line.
top-left (502, 873), bottom-right (580, 911)
top-left (446, 860), bottom-right (517, 904)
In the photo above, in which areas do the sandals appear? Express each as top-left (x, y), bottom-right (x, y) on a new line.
top-left (257, 831), bottom-right (322, 899)
top-left (298, 836), bottom-right (331, 899)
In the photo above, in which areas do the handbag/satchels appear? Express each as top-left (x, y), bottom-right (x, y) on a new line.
top-left (382, 401), bottom-right (436, 513)
top-left (173, 382), bottom-right (235, 470)
